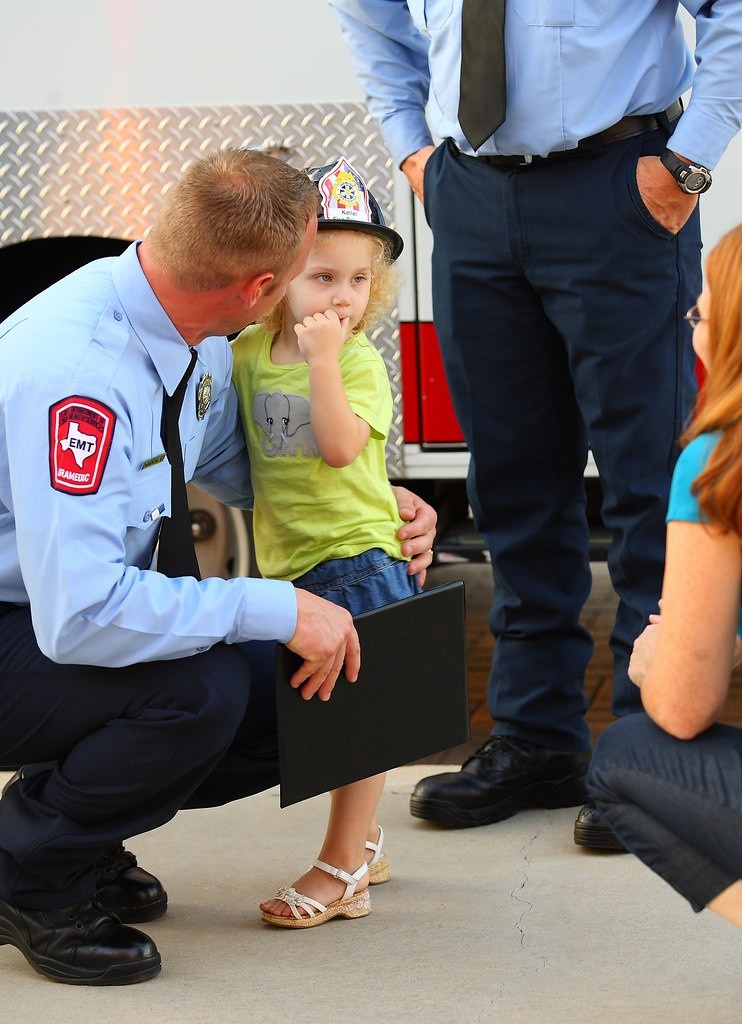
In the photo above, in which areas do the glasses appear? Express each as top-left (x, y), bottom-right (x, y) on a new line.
top-left (683, 304), bottom-right (711, 327)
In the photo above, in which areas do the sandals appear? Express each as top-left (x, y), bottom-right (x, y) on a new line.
top-left (365, 826), bottom-right (389, 883)
top-left (261, 860), bottom-right (372, 928)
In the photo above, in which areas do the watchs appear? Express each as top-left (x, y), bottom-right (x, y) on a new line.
top-left (661, 149), bottom-right (712, 193)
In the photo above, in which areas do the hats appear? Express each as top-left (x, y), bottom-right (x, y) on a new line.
top-left (309, 157), bottom-right (405, 266)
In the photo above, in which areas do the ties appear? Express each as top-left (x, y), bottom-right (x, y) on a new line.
top-left (458, 0), bottom-right (506, 152)
top-left (157, 348), bottom-right (202, 580)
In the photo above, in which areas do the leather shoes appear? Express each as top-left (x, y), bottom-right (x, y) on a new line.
top-left (574, 802), bottom-right (625, 851)
top-left (411, 735), bottom-right (592, 827)
top-left (94, 843), bottom-right (168, 922)
top-left (0, 900), bottom-right (161, 986)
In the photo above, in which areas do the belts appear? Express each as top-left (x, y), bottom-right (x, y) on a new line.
top-left (478, 96), bottom-right (685, 170)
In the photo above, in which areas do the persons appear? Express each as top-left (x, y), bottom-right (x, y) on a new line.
top-left (0, 149), bottom-right (436, 984)
top-left (584, 224), bottom-right (742, 928)
top-left (228, 157), bottom-right (424, 927)
top-left (343, 0), bottom-right (742, 850)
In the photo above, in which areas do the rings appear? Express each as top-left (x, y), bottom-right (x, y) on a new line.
top-left (428, 549), bottom-right (434, 556)
